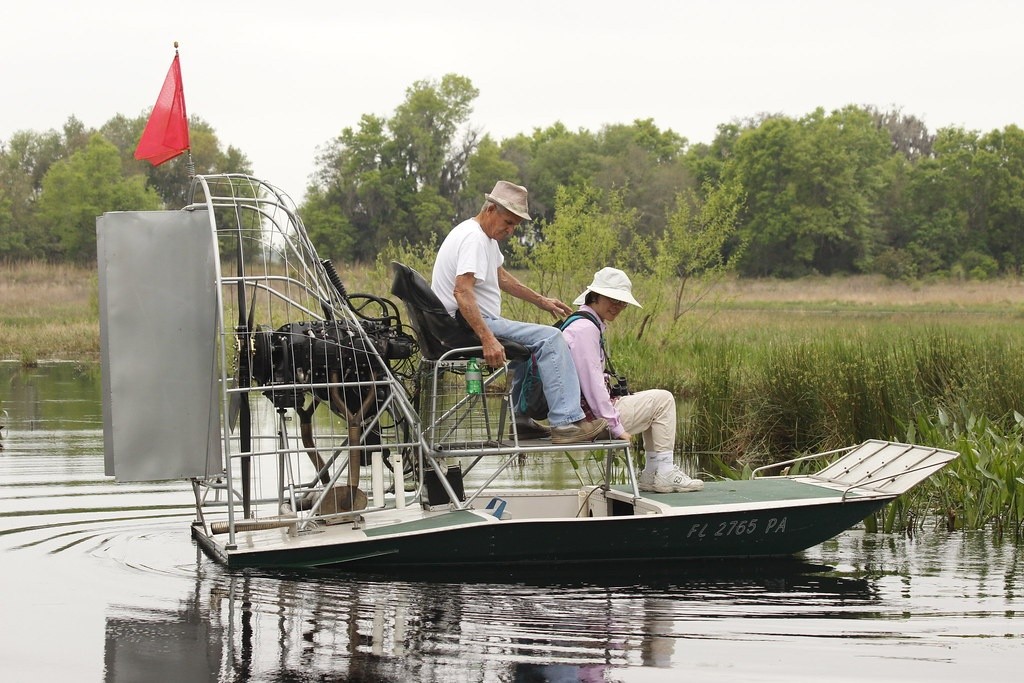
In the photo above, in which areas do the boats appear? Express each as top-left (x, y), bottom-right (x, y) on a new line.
top-left (96, 39), bottom-right (961, 577)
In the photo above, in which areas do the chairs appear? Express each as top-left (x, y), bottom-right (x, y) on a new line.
top-left (390, 261), bottom-right (530, 450)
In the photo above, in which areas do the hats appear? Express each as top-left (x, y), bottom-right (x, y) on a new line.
top-left (485, 180), bottom-right (532, 220)
top-left (573, 267), bottom-right (643, 310)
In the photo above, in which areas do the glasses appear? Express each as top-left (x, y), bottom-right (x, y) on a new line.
top-left (610, 298), bottom-right (628, 307)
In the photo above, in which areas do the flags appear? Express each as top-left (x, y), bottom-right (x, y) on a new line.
top-left (133, 53), bottom-right (191, 167)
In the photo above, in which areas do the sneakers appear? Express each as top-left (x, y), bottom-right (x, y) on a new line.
top-left (653, 465), bottom-right (703, 492)
top-left (637, 469), bottom-right (656, 492)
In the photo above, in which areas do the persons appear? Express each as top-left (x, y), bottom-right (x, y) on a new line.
top-left (431, 181), bottom-right (607, 445)
top-left (552, 266), bottom-right (706, 495)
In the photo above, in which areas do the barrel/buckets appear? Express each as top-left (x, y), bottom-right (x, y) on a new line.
top-left (423, 461), bottom-right (466, 506)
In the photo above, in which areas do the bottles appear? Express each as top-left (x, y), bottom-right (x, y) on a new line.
top-left (466, 356), bottom-right (482, 394)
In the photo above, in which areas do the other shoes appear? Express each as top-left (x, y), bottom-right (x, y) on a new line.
top-left (509, 419), bottom-right (551, 440)
top-left (551, 418), bottom-right (607, 444)
